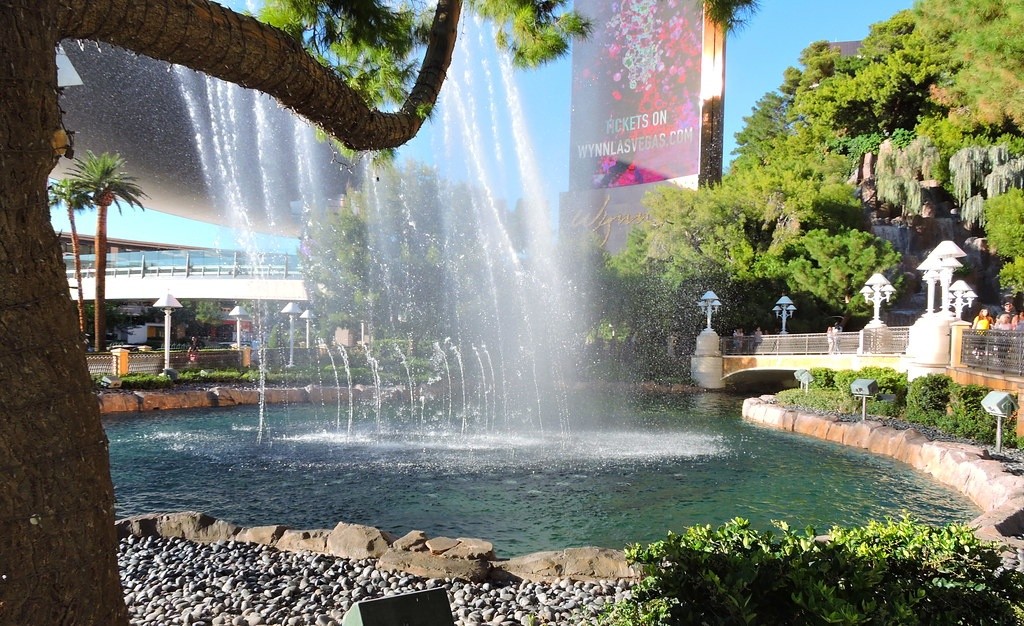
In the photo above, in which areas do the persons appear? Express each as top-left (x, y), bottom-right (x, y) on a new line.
top-left (972, 302), bottom-right (1024, 373)
top-left (827, 322), bottom-right (843, 355)
top-left (188, 336), bottom-right (198, 352)
top-left (732, 327), bottom-right (770, 355)
